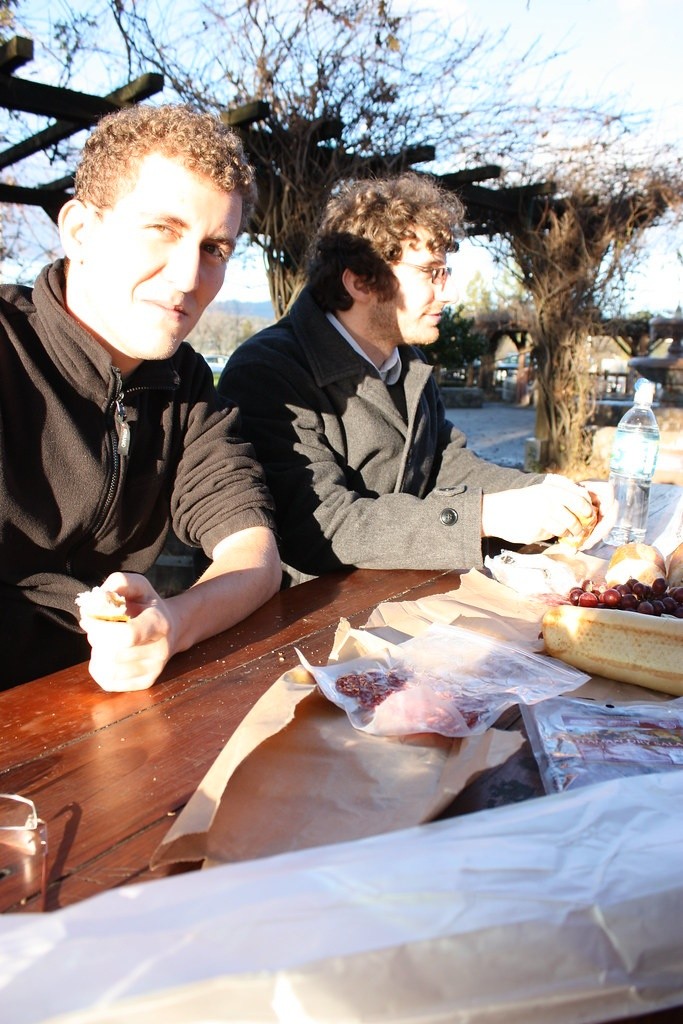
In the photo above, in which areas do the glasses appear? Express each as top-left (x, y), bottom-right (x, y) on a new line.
top-left (391, 258), bottom-right (453, 285)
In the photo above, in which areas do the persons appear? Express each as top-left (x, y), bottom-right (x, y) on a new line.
top-left (0, 106), bottom-right (283, 694)
top-left (216, 174), bottom-right (601, 570)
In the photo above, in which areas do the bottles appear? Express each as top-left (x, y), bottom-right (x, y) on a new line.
top-left (601, 378), bottom-right (661, 546)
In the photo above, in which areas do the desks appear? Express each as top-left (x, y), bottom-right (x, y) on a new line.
top-left (0, 569), bottom-right (674, 915)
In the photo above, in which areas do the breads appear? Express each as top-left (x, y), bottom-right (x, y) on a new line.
top-left (604, 541), bottom-right (683, 591)
top-left (557, 505), bottom-right (599, 548)
top-left (74, 585), bottom-right (135, 621)
